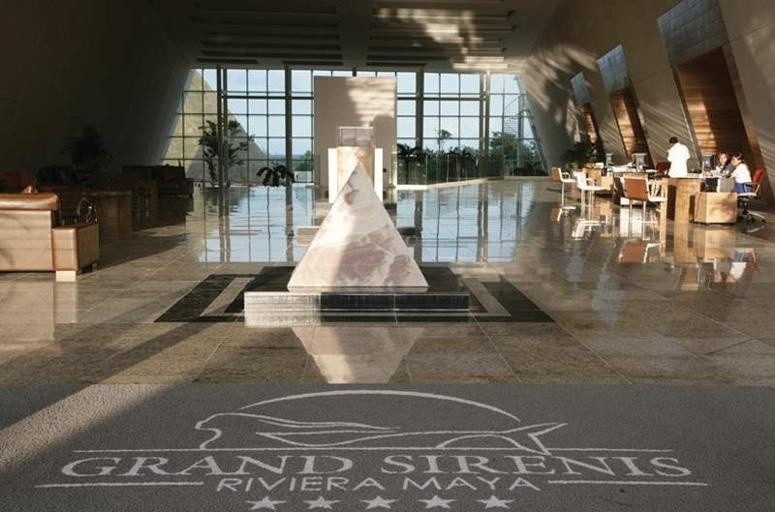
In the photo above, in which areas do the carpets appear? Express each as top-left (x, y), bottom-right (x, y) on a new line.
top-left (0, 381), bottom-right (774, 511)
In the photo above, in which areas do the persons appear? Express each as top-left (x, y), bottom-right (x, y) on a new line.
top-left (666, 136), bottom-right (691, 177)
top-left (730, 152), bottom-right (752, 194)
top-left (705, 151), bottom-right (734, 177)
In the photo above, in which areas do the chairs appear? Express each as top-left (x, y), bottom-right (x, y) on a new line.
top-left (1, 172), bottom-right (103, 284)
top-left (550, 164), bottom-right (667, 224)
top-left (738, 167), bottom-right (768, 226)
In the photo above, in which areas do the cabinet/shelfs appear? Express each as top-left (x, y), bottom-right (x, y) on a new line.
top-left (694, 190), bottom-right (738, 226)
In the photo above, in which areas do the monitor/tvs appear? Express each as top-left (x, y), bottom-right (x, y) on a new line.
top-left (702, 152), bottom-right (717, 170)
top-left (632, 153), bottom-right (650, 168)
